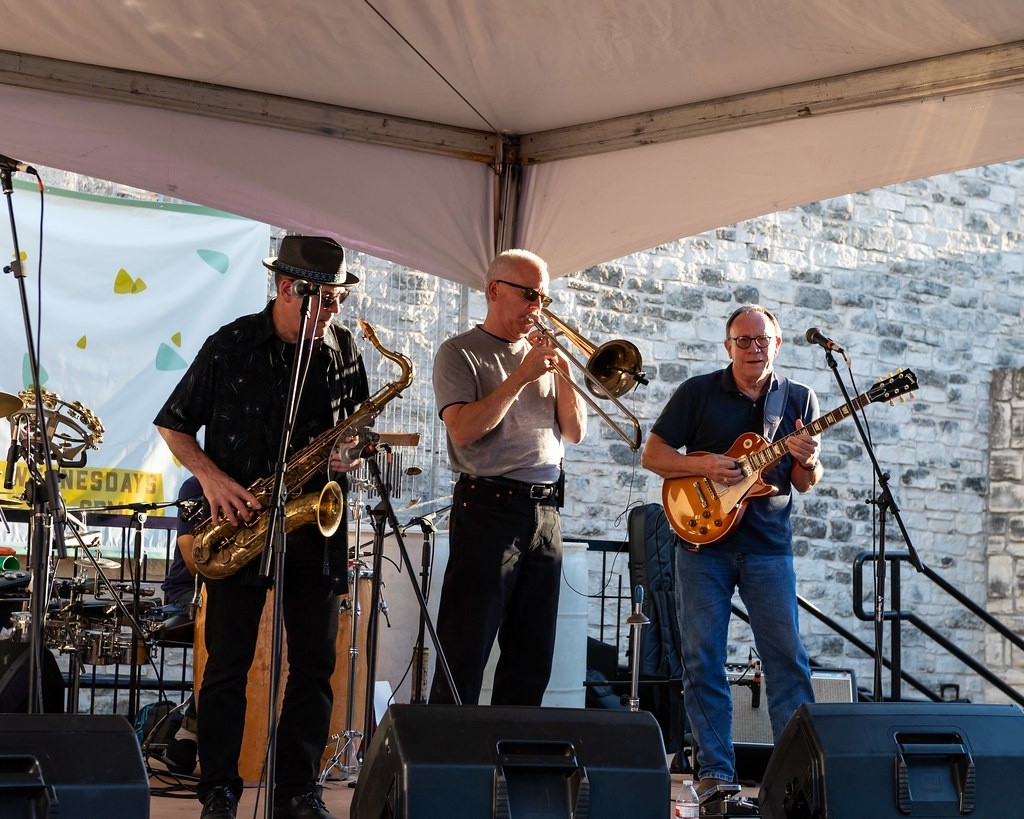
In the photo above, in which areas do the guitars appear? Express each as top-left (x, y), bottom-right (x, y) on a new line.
top-left (662, 364), bottom-right (920, 547)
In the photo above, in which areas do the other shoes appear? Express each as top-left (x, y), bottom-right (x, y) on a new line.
top-left (696, 778), bottom-right (728, 796)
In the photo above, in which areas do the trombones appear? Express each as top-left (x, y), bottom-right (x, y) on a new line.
top-left (522, 305), bottom-right (645, 452)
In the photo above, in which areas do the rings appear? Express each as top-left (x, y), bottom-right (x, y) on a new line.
top-left (724, 476), bottom-right (729, 483)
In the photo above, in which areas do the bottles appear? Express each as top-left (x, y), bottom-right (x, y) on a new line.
top-left (675, 780), bottom-right (699, 819)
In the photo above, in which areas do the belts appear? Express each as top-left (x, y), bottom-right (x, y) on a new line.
top-left (461, 470), bottom-right (559, 499)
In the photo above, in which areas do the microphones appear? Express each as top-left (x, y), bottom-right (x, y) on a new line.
top-left (290, 279), bottom-right (320, 297)
top-left (751, 661), bottom-right (760, 708)
top-left (4, 417), bottom-right (20, 489)
top-left (339, 444), bottom-right (379, 464)
top-left (805, 327), bottom-right (844, 352)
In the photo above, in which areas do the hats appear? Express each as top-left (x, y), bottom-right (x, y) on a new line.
top-left (262, 235), bottom-right (359, 286)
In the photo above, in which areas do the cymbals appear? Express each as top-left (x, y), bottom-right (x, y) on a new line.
top-left (0, 392), bottom-right (25, 419)
top-left (74, 557), bottom-right (121, 569)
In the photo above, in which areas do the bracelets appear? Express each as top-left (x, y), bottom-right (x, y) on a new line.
top-left (798, 459), bottom-right (819, 471)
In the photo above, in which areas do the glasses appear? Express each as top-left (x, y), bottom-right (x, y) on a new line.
top-left (310, 289), bottom-right (351, 306)
top-left (727, 335), bottom-right (777, 350)
top-left (496, 279), bottom-right (553, 308)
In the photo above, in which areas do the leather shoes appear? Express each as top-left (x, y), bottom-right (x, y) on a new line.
top-left (200, 785), bottom-right (237, 819)
top-left (267, 784), bottom-right (337, 819)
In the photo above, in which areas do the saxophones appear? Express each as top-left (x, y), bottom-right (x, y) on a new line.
top-left (191, 319), bottom-right (415, 582)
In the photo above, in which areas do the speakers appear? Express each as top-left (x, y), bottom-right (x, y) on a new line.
top-left (0, 714), bottom-right (150, 819)
top-left (758, 702), bottom-right (1024, 819)
top-left (350, 704), bottom-right (671, 819)
top-left (694, 663), bottom-right (858, 782)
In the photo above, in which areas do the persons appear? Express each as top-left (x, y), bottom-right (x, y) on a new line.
top-left (152, 237), bottom-right (371, 819)
top-left (641, 305), bottom-right (824, 797)
top-left (428, 247), bottom-right (588, 707)
top-left (161, 476), bottom-right (212, 774)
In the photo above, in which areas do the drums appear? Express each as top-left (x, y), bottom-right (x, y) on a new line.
top-left (12, 611), bottom-right (64, 644)
top-left (81, 629), bottom-right (119, 666)
top-left (117, 631), bottom-right (153, 665)
